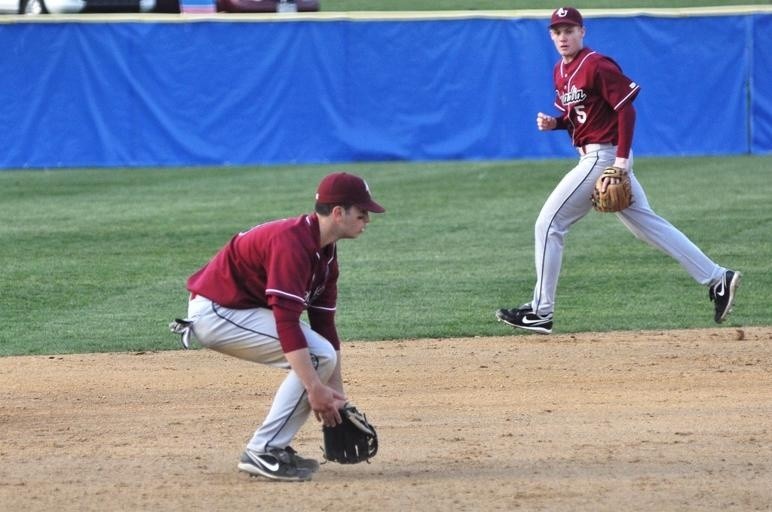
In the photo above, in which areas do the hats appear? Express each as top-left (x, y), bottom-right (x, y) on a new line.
top-left (315, 172), bottom-right (386, 213)
top-left (548, 6), bottom-right (582, 28)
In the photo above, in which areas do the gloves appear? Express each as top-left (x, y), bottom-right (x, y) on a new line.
top-left (169, 317), bottom-right (193, 349)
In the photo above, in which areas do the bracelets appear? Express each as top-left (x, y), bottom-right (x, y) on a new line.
top-left (613, 156), bottom-right (630, 170)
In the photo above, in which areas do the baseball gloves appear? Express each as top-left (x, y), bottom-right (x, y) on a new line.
top-left (323, 404), bottom-right (379, 462)
top-left (591, 168), bottom-right (633, 211)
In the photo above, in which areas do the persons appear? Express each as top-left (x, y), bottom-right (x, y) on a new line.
top-left (167, 170), bottom-right (388, 485)
top-left (494, 7), bottom-right (744, 336)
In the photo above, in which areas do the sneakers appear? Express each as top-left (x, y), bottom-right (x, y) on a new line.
top-left (708, 270), bottom-right (743, 323)
top-left (496, 302), bottom-right (553, 334)
top-left (238, 446), bottom-right (319, 482)
